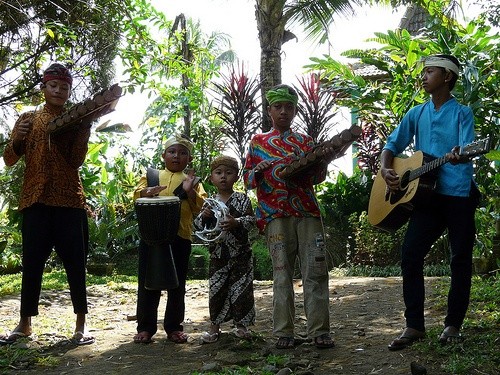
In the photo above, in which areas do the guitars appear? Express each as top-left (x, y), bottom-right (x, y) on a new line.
top-left (367, 137), bottom-right (496, 234)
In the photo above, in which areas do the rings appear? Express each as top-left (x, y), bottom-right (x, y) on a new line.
top-left (101, 114), bottom-right (103, 116)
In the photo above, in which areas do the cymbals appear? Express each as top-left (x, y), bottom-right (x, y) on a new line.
top-left (195, 197), bottom-right (231, 244)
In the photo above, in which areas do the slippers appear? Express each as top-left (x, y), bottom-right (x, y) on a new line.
top-left (200, 328), bottom-right (221, 343)
top-left (74, 328), bottom-right (95, 345)
top-left (235, 325), bottom-right (251, 338)
top-left (0, 326), bottom-right (33, 343)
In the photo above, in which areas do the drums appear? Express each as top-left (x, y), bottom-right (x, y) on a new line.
top-left (135, 196), bottom-right (182, 247)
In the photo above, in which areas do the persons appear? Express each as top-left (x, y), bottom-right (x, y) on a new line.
top-left (193, 156), bottom-right (256, 342)
top-left (0, 63), bottom-right (106, 344)
top-left (133, 132), bottom-right (207, 343)
top-left (380, 54), bottom-right (483, 351)
top-left (243, 85), bottom-right (337, 349)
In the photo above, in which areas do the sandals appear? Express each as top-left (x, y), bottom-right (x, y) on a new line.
top-left (168, 330), bottom-right (188, 343)
top-left (275, 336), bottom-right (295, 350)
top-left (314, 333), bottom-right (335, 349)
top-left (387, 327), bottom-right (427, 351)
top-left (439, 326), bottom-right (463, 347)
top-left (133, 331), bottom-right (152, 343)
